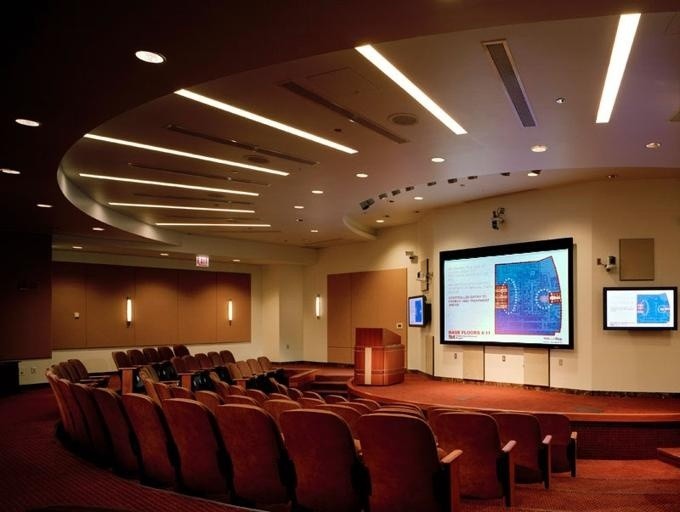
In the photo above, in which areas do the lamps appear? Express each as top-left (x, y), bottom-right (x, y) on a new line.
top-left (227, 298), bottom-right (233, 324)
top-left (125, 296), bottom-right (133, 328)
top-left (314, 291), bottom-right (320, 320)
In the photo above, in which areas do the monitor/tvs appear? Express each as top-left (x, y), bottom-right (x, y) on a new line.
top-left (603, 286), bottom-right (677, 330)
top-left (408, 295), bottom-right (431, 328)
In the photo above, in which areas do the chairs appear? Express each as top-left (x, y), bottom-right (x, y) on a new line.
top-left (46, 344), bottom-right (579, 511)
